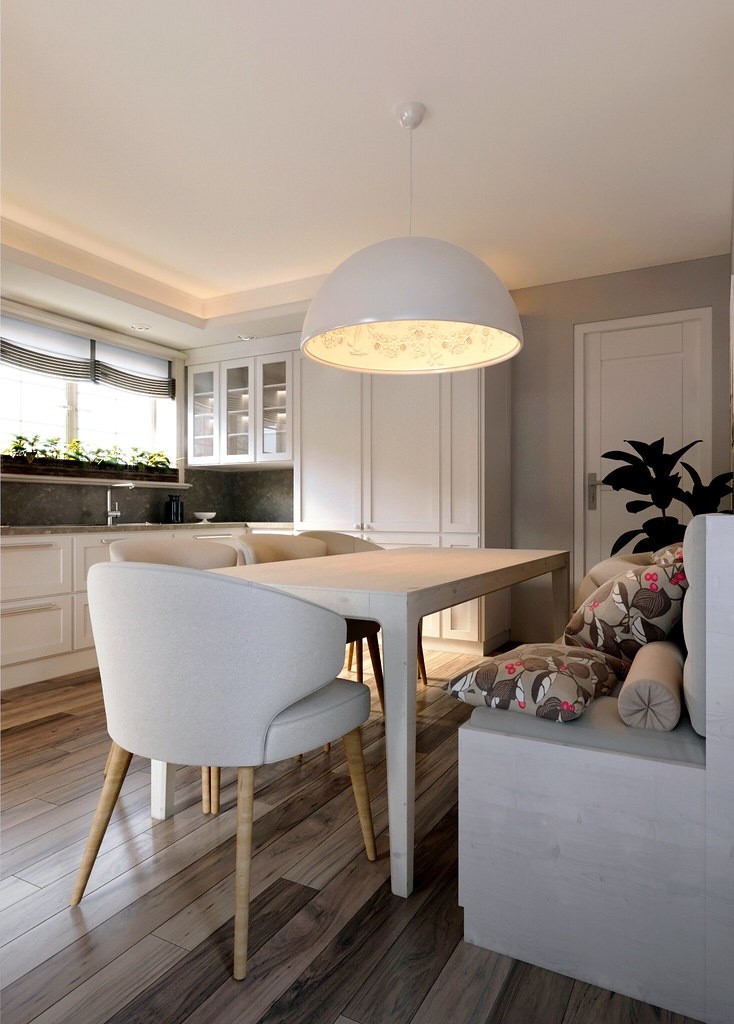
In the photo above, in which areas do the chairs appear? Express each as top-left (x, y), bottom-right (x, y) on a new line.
top-left (68, 562), bottom-right (376, 981)
top-left (233, 533), bottom-right (385, 752)
top-left (107, 537), bottom-right (303, 815)
top-left (298, 529), bottom-right (429, 686)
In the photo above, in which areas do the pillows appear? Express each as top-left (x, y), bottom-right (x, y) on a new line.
top-left (440, 643), bottom-right (632, 724)
top-left (645, 542), bottom-right (683, 565)
top-left (562, 563), bottom-right (688, 662)
top-left (616, 640), bottom-right (685, 733)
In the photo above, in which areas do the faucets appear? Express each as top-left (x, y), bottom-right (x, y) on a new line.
top-left (106, 483), bottom-right (134, 525)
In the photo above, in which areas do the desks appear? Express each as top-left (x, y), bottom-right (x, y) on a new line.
top-left (151, 545), bottom-right (571, 900)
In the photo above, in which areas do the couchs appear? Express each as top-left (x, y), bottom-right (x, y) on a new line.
top-left (458, 513), bottom-right (734, 1024)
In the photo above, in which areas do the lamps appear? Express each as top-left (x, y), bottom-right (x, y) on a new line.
top-left (299, 103), bottom-right (523, 376)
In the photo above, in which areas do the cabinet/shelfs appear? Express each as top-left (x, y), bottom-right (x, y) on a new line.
top-left (0, 529), bottom-right (120, 693)
top-left (187, 352), bottom-right (294, 473)
top-left (293, 349), bottom-right (513, 657)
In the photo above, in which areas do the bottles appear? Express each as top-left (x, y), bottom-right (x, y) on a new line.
top-left (165, 494), bottom-right (184, 523)
